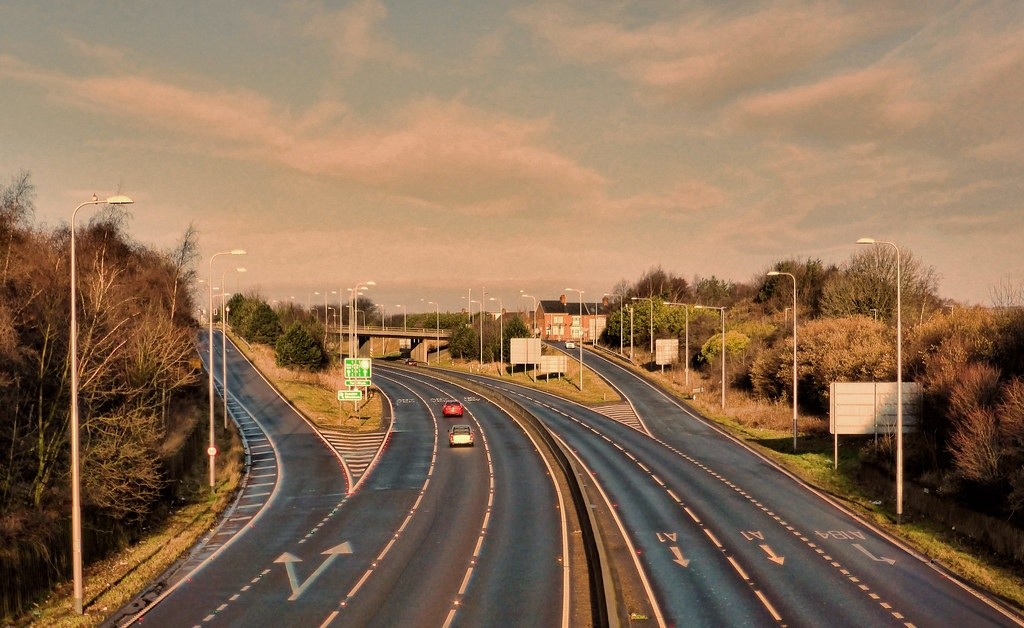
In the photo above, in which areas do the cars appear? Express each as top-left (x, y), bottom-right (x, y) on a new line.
top-left (441, 400), bottom-right (464, 418)
top-left (447, 425), bottom-right (477, 448)
top-left (404, 358), bottom-right (418, 367)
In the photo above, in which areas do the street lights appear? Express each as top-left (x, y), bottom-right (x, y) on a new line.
top-left (221, 268), bottom-right (248, 432)
top-left (314, 291), bottom-right (328, 328)
top-left (429, 301), bottom-right (439, 365)
top-left (522, 295), bottom-right (536, 383)
top-left (208, 249), bottom-right (249, 493)
top-left (604, 293), bottom-right (624, 356)
top-left (70, 195), bottom-right (138, 615)
top-left (331, 288), bottom-right (343, 363)
top-left (375, 304), bottom-right (384, 331)
top-left (767, 270), bottom-right (797, 455)
top-left (566, 288), bottom-right (583, 392)
top-left (328, 307), bottom-right (336, 328)
top-left (354, 281), bottom-right (376, 359)
top-left (396, 305), bottom-right (406, 332)
top-left (694, 305), bottom-right (726, 410)
top-left (354, 310), bottom-right (366, 329)
top-left (346, 306), bottom-right (354, 326)
top-left (489, 297), bottom-right (503, 376)
top-left (662, 302), bottom-right (689, 386)
top-left (630, 297), bottom-right (654, 371)
top-left (471, 300), bottom-right (483, 365)
top-left (855, 238), bottom-right (904, 525)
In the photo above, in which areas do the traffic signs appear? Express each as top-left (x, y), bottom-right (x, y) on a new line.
top-left (345, 379), bottom-right (372, 386)
top-left (338, 390), bottom-right (362, 401)
top-left (344, 357), bottom-right (371, 378)
